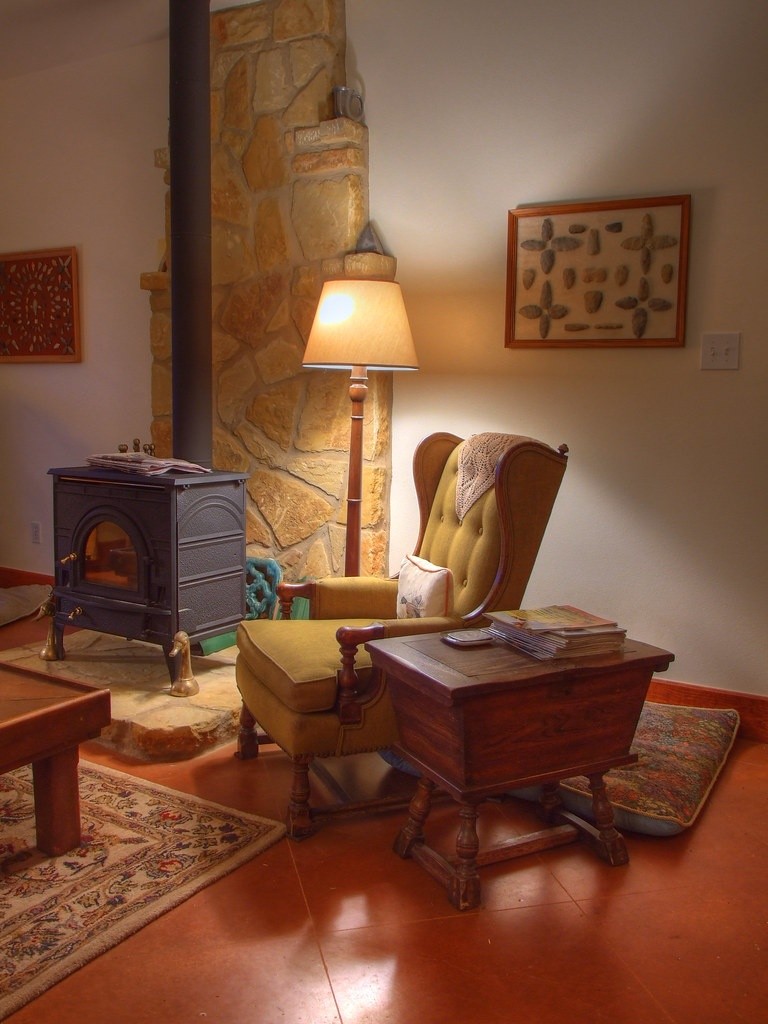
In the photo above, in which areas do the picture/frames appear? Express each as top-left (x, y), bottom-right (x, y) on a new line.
top-left (0, 245), bottom-right (81, 364)
top-left (505, 194), bottom-right (691, 348)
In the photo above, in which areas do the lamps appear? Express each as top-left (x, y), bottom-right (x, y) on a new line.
top-left (302, 280), bottom-right (418, 577)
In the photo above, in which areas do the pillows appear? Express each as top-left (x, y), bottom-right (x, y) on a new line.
top-left (397, 554), bottom-right (454, 619)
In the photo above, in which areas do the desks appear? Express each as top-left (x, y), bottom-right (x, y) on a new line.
top-left (364, 629), bottom-right (675, 912)
top-left (0, 659), bottom-right (111, 857)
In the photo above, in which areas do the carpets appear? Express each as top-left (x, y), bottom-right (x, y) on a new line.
top-left (0, 758), bottom-right (287, 1020)
top-left (377, 700), bottom-right (739, 838)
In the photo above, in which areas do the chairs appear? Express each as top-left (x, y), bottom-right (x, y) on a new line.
top-left (235, 431), bottom-right (569, 842)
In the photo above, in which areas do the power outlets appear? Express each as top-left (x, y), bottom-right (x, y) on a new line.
top-left (699, 331), bottom-right (739, 370)
